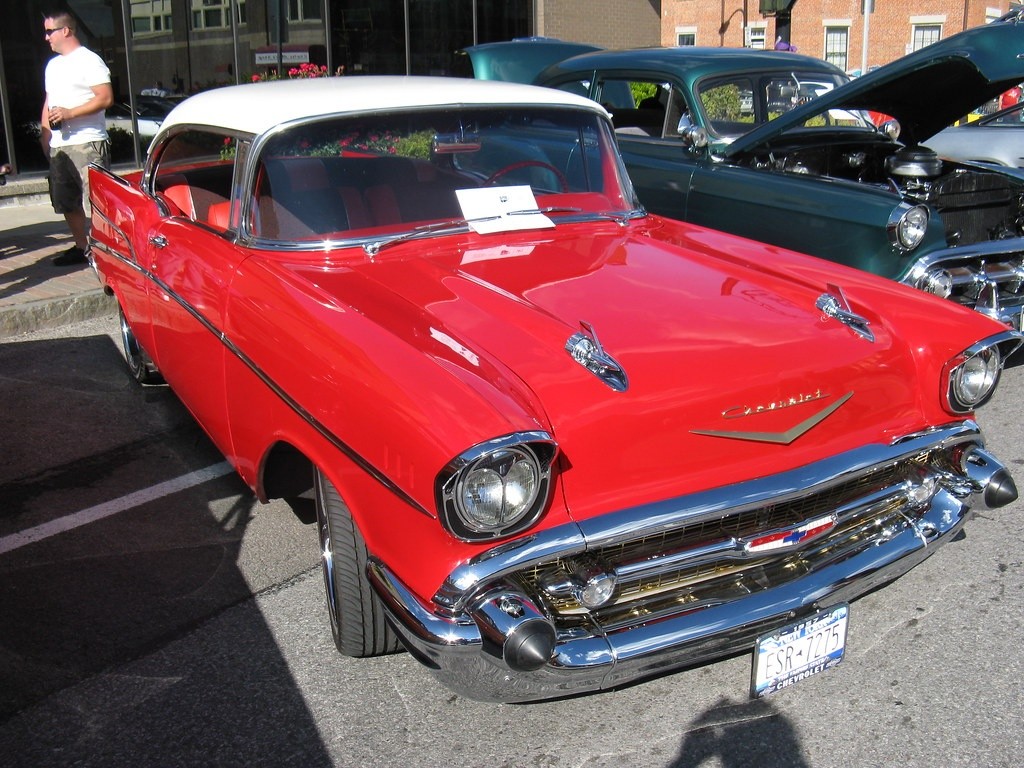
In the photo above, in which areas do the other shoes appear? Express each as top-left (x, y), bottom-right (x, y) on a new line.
top-left (54, 246), bottom-right (88, 265)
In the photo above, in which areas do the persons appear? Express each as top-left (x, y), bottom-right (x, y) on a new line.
top-left (41, 12), bottom-right (115, 266)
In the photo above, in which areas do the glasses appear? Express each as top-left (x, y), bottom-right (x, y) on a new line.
top-left (41, 27), bottom-right (73, 37)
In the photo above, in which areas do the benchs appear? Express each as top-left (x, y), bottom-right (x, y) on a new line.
top-left (600, 97), bottom-right (666, 137)
top-left (156, 155), bottom-right (439, 242)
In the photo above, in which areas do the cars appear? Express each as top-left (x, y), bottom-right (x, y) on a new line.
top-left (108, 89), bottom-right (191, 122)
top-left (791, 81), bottom-right (859, 121)
top-left (82, 74), bottom-right (1023, 703)
top-left (918, 101), bottom-right (1024, 171)
top-left (766, 96), bottom-right (792, 112)
top-left (736, 89), bottom-right (755, 114)
top-left (447, 4), bottom-right (1024, 347)
top-left (869, 110), bottom-right (901, 136)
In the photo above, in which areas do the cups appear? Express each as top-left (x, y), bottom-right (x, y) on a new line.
top-left (48, 106), bottom-right (62, 130)
